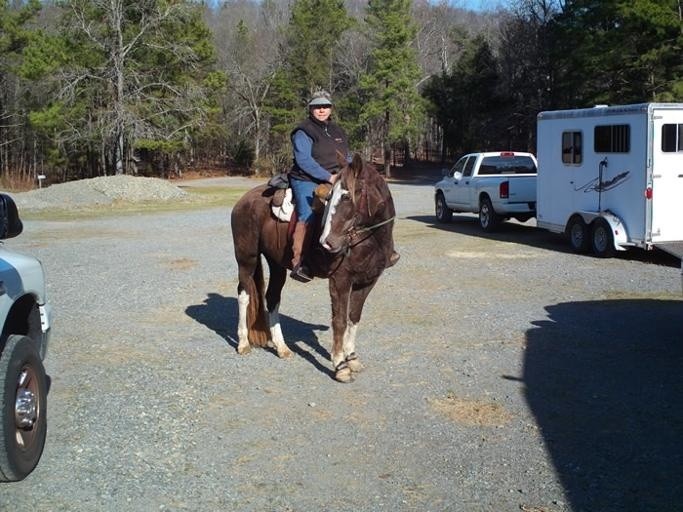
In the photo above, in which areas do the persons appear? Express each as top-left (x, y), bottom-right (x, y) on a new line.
top-left (287, 89), bottom-right (353, 283)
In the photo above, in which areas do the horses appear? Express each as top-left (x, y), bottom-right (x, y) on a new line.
top-left (231, 148), bottom-right (396, 384)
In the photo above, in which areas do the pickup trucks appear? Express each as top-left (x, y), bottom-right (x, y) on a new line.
top-left (434, 151), bottom-right (538, 231)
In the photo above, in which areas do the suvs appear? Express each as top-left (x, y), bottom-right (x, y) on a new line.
top-left (1, 194), bottom-right (52, 482)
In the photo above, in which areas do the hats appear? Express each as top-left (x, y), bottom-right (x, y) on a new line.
top-left (308, 89), bottom-right (333, 108)
top-left (272, 188), bottom-right (294, 222)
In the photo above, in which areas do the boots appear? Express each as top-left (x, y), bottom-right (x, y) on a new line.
top-left (291, 221), bottom-right (315, 282)
